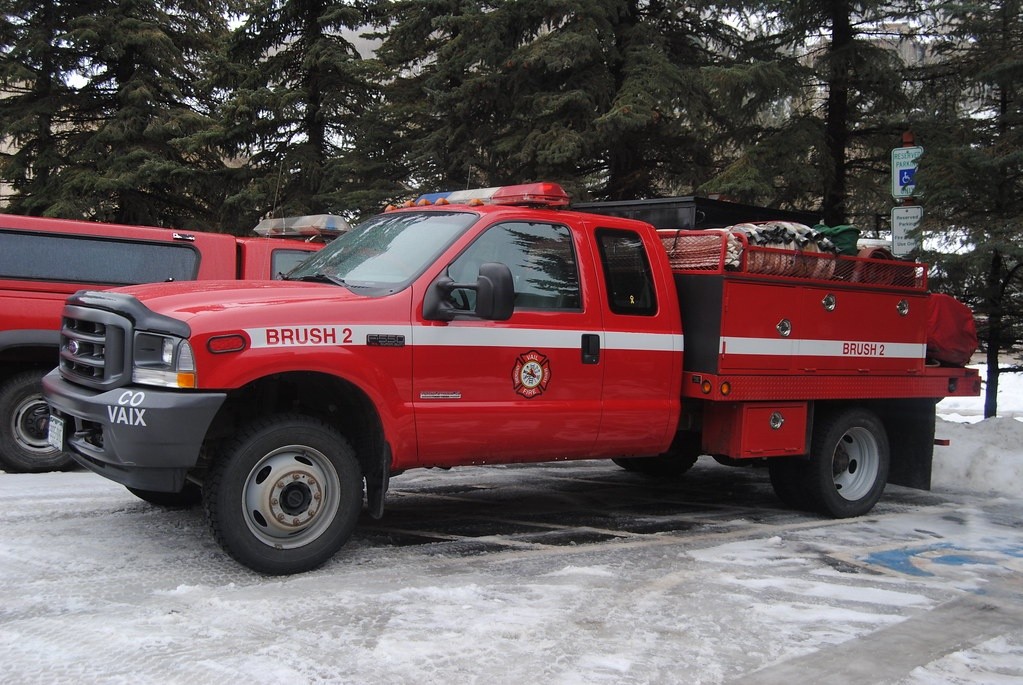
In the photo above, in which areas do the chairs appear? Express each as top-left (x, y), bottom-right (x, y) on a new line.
top-left (451, 232), bottom-right (505, 312)
top-left (515, 254), bottom-right (570, 308)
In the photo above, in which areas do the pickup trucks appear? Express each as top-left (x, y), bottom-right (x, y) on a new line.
top-left (40, 180), bottom-right (982, 577)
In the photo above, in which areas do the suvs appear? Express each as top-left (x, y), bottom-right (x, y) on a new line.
top-left (0, 214), bottom-right (354, 474)
